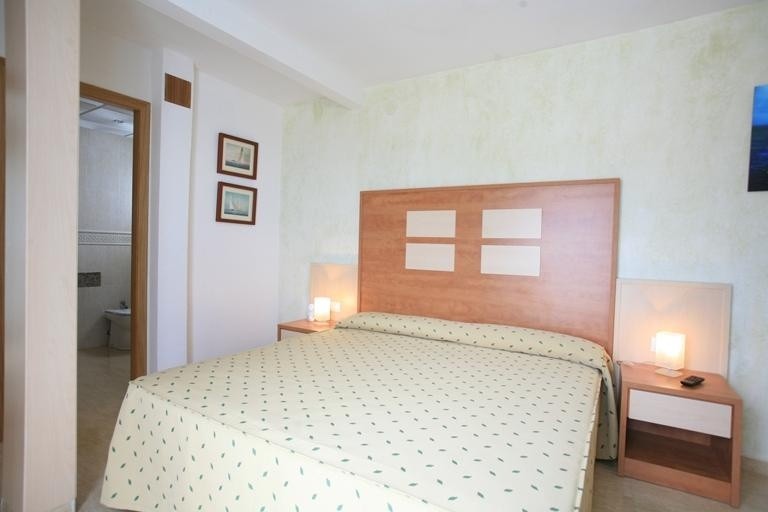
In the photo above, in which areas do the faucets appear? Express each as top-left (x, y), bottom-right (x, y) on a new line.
top-left (120, 301), bottom-right (128, 309)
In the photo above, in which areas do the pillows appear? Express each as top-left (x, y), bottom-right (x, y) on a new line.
top-left (339, 311), bottom-right (605, 372)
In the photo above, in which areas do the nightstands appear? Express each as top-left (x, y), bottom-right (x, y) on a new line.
top-left (616, 362), bottom-right (743, 508)
top-left (277, 318), bottom-right (338, 342)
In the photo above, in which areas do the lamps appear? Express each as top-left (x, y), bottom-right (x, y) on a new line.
top-left (314, 296), bottom-right (331, 326)
top-left (653, 331), bottom-right (685, 378)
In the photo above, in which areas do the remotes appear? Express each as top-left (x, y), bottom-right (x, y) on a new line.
top-left (680, 375), bottom-right (704, 386)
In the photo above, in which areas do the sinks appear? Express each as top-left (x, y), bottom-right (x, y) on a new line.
top-left (105, 309), bottom-right (132, 316)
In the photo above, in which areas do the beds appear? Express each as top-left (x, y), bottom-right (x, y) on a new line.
top-left (127, 178), bottom-right (621, 512)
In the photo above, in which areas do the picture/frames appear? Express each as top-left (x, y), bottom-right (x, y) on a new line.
top-left (216, 132), bottom-right (258, 180)
top-left (216, 182), bottom-right (257, 225)
